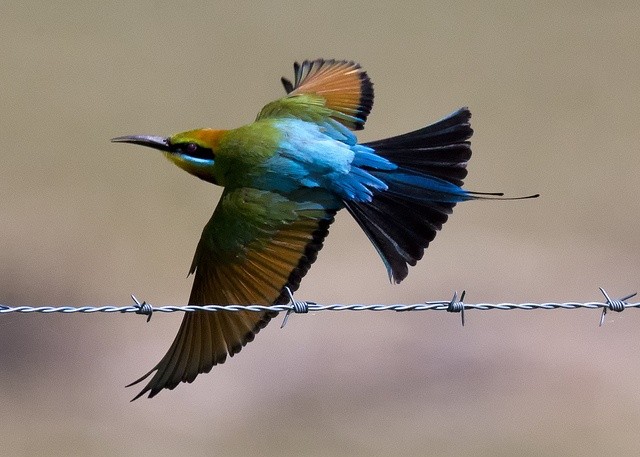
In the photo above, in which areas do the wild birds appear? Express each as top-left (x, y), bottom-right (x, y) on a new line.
top-left (111, 58), bottom-right (539, 405)
